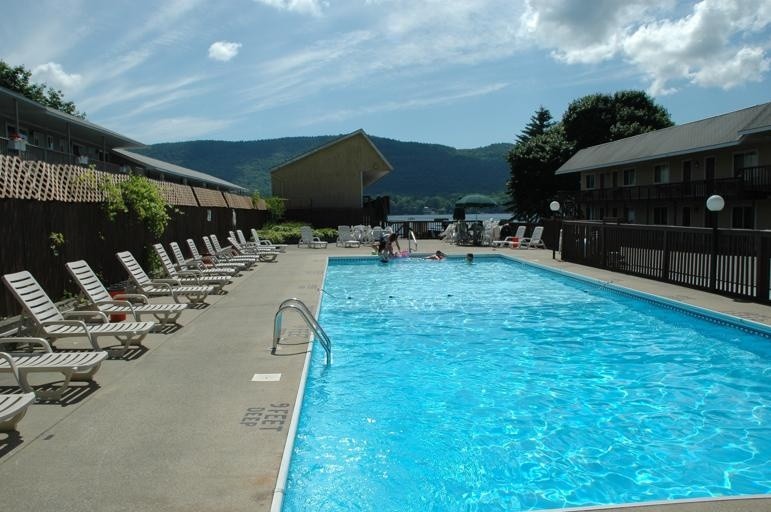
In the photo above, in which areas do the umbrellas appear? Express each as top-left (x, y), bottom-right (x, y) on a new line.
top-left (456, 193), bottom-right (498, 219)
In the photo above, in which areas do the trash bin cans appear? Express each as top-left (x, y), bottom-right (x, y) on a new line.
top-left (513, 237), bottom-right (518, 248)
top-left (106, 286), bottom-right (126, 321)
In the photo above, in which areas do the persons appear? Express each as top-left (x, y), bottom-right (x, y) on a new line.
top-left (378, 233), bottom-right (401, 263)
top-left (465, 253), bottom-right (474, 264)
top-left (426, 250), bottom-right (448, 260)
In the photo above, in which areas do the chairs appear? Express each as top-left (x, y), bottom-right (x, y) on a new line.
top-left (298, 225), bottom-right (394, 249)
top-left (2, 336), bottom-right (108, 399)
top-left (440, 219), bottom-right (546, 250)
top-left (66, 260), bottom-right (188, 332)
top-left (3, 270), bottom-right (155, 357)
top-left (152, 230), bottom-right (289, 293)
top-left (1, 392), bottom-right (36, 433)
top-left (117, 251), bottom-right (215, 307)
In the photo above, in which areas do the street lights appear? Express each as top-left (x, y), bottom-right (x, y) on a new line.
top-left (550, 201), bottom-right (560, 259)
top-left (706, 195), bottom-right (724, 289)
